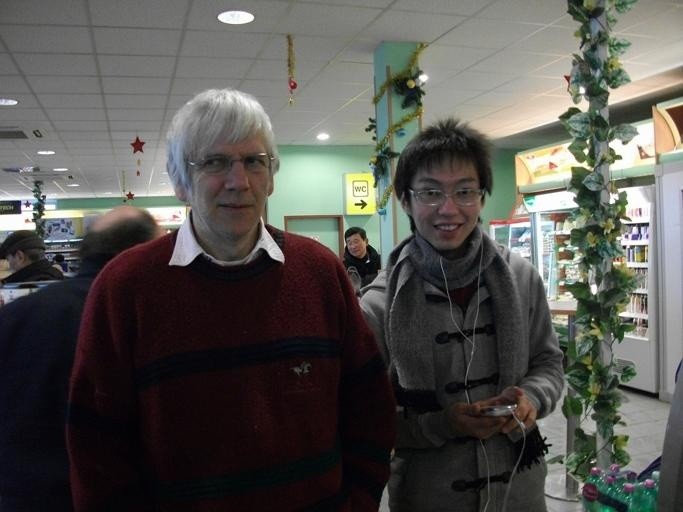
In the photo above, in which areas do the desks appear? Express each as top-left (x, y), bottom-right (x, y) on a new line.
top-left (543, 300), bottom-right (618, 503)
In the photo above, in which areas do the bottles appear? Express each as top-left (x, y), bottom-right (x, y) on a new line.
top-left (626, 269), bottom-right (647, 315)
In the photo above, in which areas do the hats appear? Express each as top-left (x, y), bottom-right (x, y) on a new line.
top-left (0, 230), bottom-right (46, 259)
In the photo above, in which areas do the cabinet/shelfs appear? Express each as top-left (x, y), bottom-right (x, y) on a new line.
top-left (489, 203), bottom-right (659, 394)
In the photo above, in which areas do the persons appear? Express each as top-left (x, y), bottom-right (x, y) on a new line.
top-left (1, 206), bottom-right (164, 512)
top-left (67, 88), bottom-right (395, 512)
top-left (337, 226), bottom-right (381, 293)
top-left (0, 230), bottom-right (65, 281)
top-left (357, 116), bottom-right (563, 511)
top-left (54, 255), bottom-right (69, 273)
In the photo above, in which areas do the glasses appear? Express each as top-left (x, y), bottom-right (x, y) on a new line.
top-left (408, 188), bottom-right (483, 206)
top-left (186, 153), bottom-right (274, 174)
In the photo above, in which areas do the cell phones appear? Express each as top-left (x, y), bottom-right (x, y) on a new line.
top-left (479, 403), bottom-right (517, 418)
